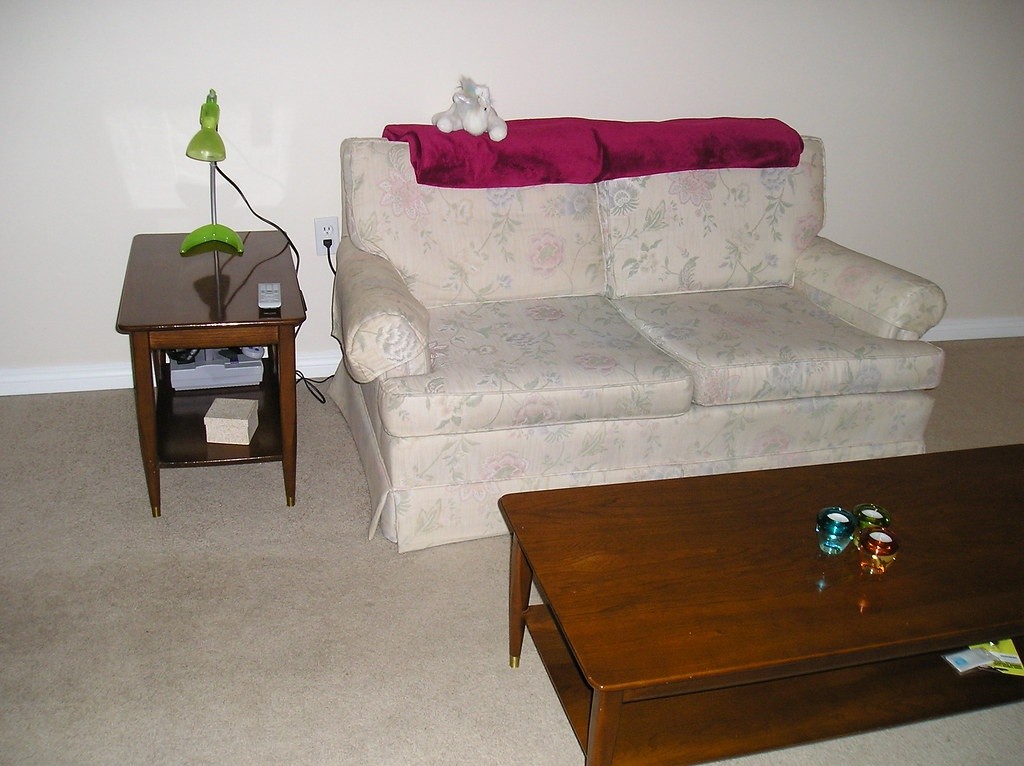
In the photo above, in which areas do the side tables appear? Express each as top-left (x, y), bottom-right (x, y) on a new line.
top-left (115, 231), bottom-right (307, 517)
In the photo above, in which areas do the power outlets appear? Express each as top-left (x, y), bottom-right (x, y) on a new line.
top-left (315, 216), bottom-right (340, 256)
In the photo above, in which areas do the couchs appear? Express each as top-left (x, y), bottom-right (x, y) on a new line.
top-left (327, 137), bottom-right (946, 554)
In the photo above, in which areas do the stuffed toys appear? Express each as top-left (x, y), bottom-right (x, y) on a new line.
top-left (431, 76), bottom-right (508, 142)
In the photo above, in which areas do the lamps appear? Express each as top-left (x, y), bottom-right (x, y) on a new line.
top-left (179, 88), bottom-right (244, 253)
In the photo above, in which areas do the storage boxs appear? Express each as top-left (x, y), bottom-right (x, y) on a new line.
top-left (204, 398), bottom-right (259, 445)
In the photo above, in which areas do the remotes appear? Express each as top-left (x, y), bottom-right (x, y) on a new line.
top-left (258, 282), bottom-right (282, 312)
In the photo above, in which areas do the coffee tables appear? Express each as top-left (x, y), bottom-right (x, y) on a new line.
top-left (498, 443), bottom-right (1024, 766)
